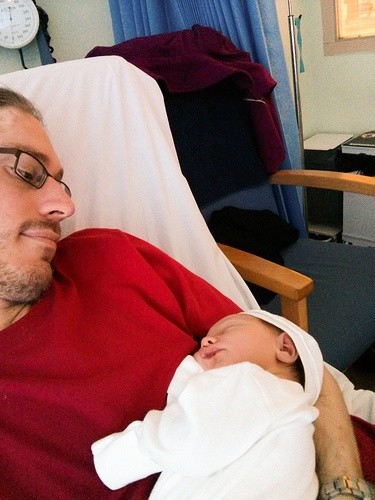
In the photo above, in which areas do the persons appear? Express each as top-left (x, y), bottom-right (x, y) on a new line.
top-left (90, 309), bottom-right (323, 500)
top-left (0, 88), bottom-right (375, 500)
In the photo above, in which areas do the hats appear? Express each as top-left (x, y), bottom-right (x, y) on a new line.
top-left (238, 309), bottom-right (323, 405)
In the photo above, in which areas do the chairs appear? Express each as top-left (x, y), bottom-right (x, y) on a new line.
top-left (84, 29), bottom-right (375, 385)
top-left (0, 55), bottom-right (374, 500)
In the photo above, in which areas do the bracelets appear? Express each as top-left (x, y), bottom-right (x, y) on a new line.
top-left (316, 475), bottom-right (375, 500)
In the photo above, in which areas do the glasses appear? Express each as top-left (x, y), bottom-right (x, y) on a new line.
top-left (0, 147), bottom-right (72, 198)
